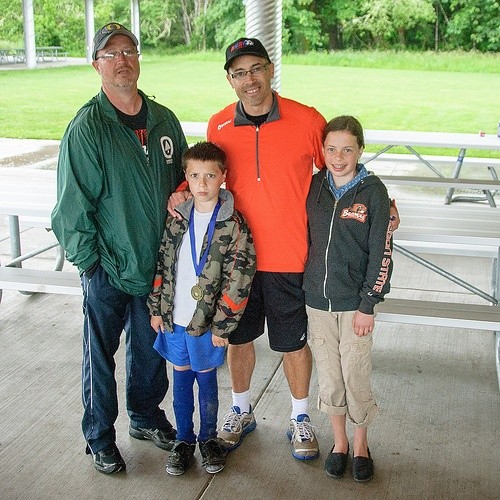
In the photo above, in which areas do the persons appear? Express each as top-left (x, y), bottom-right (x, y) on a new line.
top-left (303, 116), bottom-right (393, 482)
top-left (168, 38), bottom-right (400, 460)
top-left (147, 141), bottom-right (256, 476)
top-left (51, 23), bottom-right (191, 476)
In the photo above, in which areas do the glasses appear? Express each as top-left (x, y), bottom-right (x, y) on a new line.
top-left (227, 61), bottom-right (269, 80)
top-left (96, 48), bottom-right (140, 63)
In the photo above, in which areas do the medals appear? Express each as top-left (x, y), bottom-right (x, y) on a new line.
top-left (190, 285), bottom-right (204, 301)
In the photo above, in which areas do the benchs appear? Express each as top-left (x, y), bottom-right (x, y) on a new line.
top-left (0, 148), bottom-right (500, 333)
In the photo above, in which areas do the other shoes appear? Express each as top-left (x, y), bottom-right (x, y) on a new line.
top-left (352, 446), bottom-right (375, 483)
top-left (199, 435), bottom-right (226, 474)
top-left (165, 440), bottom-right (196, 475)
top-left (324, 442), bottom-right (350, 480)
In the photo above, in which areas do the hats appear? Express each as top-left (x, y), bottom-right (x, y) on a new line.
top-left (224, 38), bottom-right (271, 71)
top-left (92, 22), bottom-right (138, 60)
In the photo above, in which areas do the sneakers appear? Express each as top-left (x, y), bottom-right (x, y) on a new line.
top-left (286, 414), bottom-right (319, 461)
top-left (85, 441), bottom-right (126, 474)
top-left (129, 421), bottom-right (177, 451)
top-left (217, 403), bottom-right (257, 452)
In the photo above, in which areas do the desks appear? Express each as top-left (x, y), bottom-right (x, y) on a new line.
top-left (0, 167), bottom-right (500, 260)
top-left (180, 120), bottom-right (500, 148)
